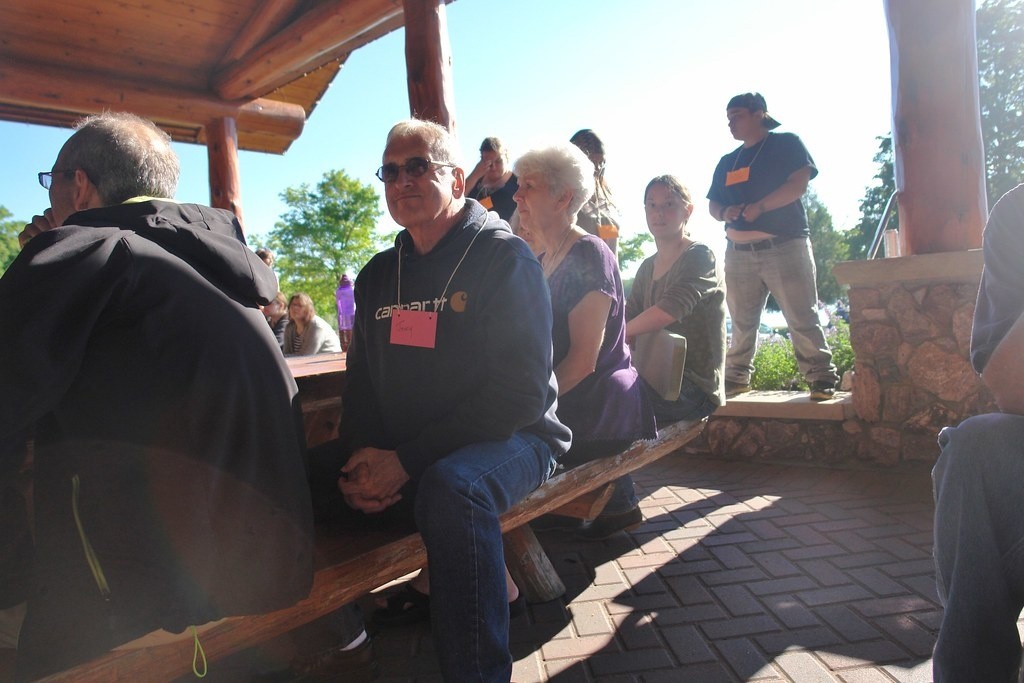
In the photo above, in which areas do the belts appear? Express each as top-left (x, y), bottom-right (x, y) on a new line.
top-left (728, 236), bottom-right (791, 251)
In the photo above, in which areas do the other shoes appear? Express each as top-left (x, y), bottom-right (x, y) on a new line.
top-left (724, 380), bottom-right (751, 395)
top-left (533, 511), bottom-right (583, 536)
top-left (809, 381), bottom-right (835, 399)
top-left (581, 506), bottom-right (641, 539)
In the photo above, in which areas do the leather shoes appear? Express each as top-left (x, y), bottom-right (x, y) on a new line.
top-left (251, 635), bottom-right (377, 682)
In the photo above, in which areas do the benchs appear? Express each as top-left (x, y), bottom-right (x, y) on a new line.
top-left (29, 409), bottom-right (712, 683)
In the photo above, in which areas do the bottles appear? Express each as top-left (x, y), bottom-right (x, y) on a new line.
top-left (336, 274), bottom-right (357, 351)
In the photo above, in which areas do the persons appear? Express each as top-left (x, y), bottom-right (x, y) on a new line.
top-left (374, 142), bottom-right (659, 626)
top-left (255, 247), bottom-right (343, 358)
top-left (254, 118), bottom-right (573, 683)
top-left (528, 174), bottom-right (727, 541)
top-left (932, 183), bottom-right (1024, 683)
top-left (568, 129), bottom-right (620, 259)
top-left (705, 92), bottom-right (841, 401)
top-left (464, 137), bottom-right (547, 264)
top-left (0, 111), bottom-right (316, 682)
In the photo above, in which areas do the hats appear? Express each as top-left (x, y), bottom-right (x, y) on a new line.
top-left (725, 91), bottom-right (782, 130)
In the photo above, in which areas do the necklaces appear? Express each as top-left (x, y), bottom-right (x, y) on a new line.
top-left (541, 223), bottom-right (575, 276)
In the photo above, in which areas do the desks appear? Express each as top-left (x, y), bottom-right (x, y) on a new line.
top-left (282, 350), bottom-right (615, 604)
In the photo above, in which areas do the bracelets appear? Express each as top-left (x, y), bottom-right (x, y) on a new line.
top-left (719, 206), bottom-right (726, 221)
top-left (757, 201), bottom-right (765, 215)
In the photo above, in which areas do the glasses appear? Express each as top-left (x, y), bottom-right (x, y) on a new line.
top-left (38, 168), bottom-right (75, 191)
top-left (375, 157), bottom-right (456, 183)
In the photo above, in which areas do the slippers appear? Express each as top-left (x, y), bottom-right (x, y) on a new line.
top-left (507, 595), bottom-right (523, 618)
top-left (372, 583), bottom-right (431, 627)
